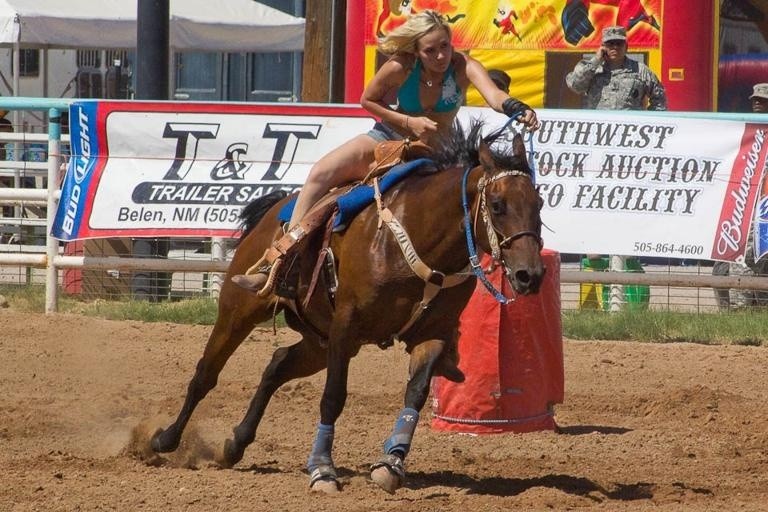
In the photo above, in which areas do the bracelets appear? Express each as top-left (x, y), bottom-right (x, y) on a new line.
top-left (400, 115), bottom-right (409, 131)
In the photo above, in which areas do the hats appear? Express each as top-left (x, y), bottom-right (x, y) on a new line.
top-left (747, 83), bottom-right (768, 101)
top-left (485, 68), bottom-right (511, 87)
top-left (600, 26), bottom-right (628, 45)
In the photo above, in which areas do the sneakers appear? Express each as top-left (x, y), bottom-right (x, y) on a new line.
top-left (433, 356), bottom-right (469, 384)
top-left (230, 257), bottom-right (303, 299)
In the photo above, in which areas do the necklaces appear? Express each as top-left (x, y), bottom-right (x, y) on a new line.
top-left (421, 68), bottom-right (434, 90)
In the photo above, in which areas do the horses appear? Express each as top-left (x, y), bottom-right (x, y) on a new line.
top-left (147, 111), bottom-right (548, 496)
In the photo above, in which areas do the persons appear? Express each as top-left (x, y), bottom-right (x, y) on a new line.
top-left (0, 109), bottom-right (14, 160)
top-left (564, 24), bottom-right (667, 112)
top-left (229, 7), bottom-right (545, 383)
top-left (486, 67), bottom-right (513, 96)
top-left (724, 79), bottom-right (768, 310)
